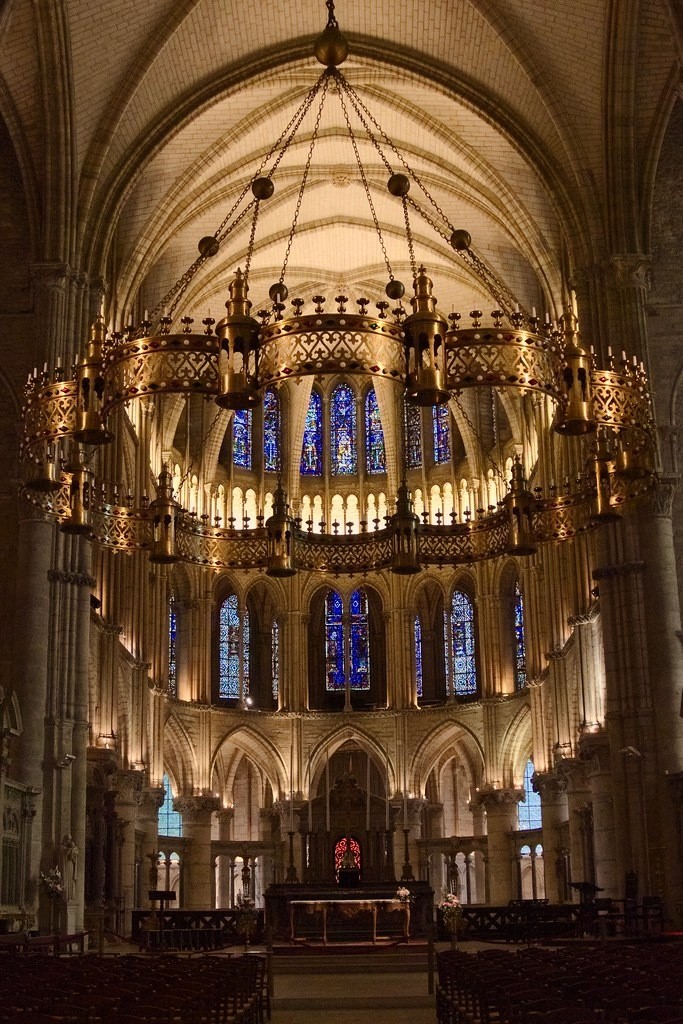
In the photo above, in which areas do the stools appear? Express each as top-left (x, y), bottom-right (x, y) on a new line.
top-left (146, 929), bottom-right (224, 952)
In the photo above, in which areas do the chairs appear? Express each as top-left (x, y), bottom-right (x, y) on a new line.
top-left (435, 944), bottom-right (683, 1024)
top-left (0, 953), bottom-right (273, 1024)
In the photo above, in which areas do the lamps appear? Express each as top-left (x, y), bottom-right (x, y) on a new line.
top-left (21, 0), bottom-right (660, 578)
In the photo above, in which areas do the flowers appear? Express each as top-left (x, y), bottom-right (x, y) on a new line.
top-left (397, 885), bottom-right (415, 900)
top-left (35, 866), bottom-right (67, 898)
top-left (438, 883), bottom-right (462, 913)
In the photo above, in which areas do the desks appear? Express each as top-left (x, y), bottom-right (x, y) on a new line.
top-left (263, 880), bottom-right (436, 939)
top-left (290, 901), bottom-right (413, 944)
